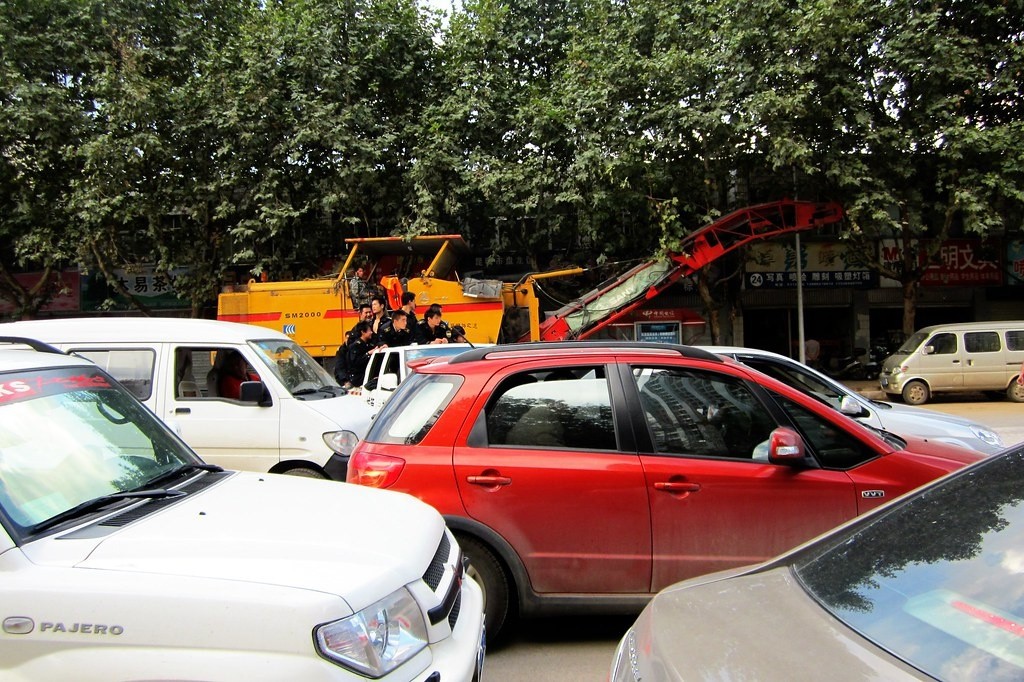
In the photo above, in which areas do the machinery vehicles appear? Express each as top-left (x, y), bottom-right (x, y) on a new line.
top-left (210, 198), bottom-right (841, 389)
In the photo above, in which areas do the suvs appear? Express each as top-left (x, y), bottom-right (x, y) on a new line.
top-left (348, 340), bottom-right (1000, 652)
top-left (0, 335), bottom-right (488, 682)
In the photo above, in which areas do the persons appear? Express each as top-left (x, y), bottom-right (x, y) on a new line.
top-left (215, 350), bottom-right (263, 398)
top-left (804, 334), bottom-right (822, 369)
top-left (334, 265), bottom-right (466, 392)
top-left (504, 369), bottom-right (581, 447)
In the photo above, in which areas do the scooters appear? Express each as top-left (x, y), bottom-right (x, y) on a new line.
top-left (818, 347), bottom-right (866, 380)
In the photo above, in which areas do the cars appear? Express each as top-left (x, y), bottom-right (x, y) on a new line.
top-left (600, 442), bottom-right (1024, 682)
top-left (587, 346), bottom-right (1010, 469)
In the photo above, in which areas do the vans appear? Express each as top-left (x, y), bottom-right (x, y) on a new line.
top-left (0, 318), bottom-right (375, 486)
top-left (879, 320), bottom-right (1024, 405)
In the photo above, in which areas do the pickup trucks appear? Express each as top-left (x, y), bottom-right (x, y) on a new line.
top-left (344, 342), bottom-right (495, 411)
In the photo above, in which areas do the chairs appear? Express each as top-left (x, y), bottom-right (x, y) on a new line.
top-left (177, 363), bottom-right (202, 398)
top-left (207, 350), bottom-right (228, 396)
